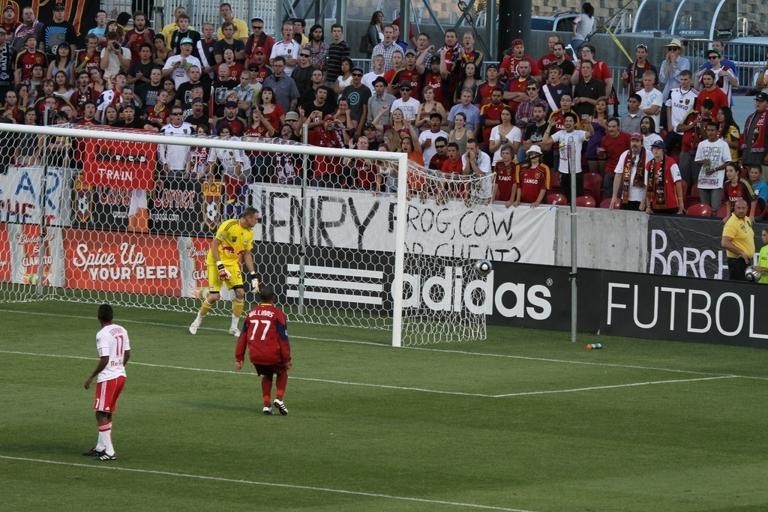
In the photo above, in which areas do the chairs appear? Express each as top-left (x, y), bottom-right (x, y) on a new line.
top-left (536, 163), bottom-right (745, 225)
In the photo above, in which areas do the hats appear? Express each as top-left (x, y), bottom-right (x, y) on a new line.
top-left (754, 92), bottom-right (767, 101)
top-left (372, 76), bottom-right (388, 87)
top-left (665, 39), bottom-right (681, 47)
top-left (650, 140), bottom-right (665, 148)
top-left (324, 114), bottom-right (334, 120)
top-left (398, 80), bottom-right (415, 89)
top-left (707, 48), bottom-right (721, 58)
top-left (254, 46), bottom-right (266, 53)
top-left (180, 37), bottom-right (192, 45)
top-left (631, 132), bottom-right (642, 140)
top-left (285, 111), bottom-right (298, 122)
top-left (526, 145), bottom-right (542, 156)
top-left (405, 49), bottom-right (416, 55)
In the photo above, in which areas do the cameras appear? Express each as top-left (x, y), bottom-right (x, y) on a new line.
top-left (112, 42), bottom-right (120, 49)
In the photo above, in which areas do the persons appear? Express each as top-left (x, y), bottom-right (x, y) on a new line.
top-left (721, 199), bottom-right (755, 280)
top-left (84, 304), bottom-right (131, 462)
top-left (753, 226), bottom-right (768, 284)
top-left (0, 0), bottom-right (768, 224)
top-left (189, 208), bottom-right (261, 337)
top-left (233, 286), bottom-right (292, 416)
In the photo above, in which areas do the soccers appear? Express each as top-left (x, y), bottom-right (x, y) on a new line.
top-left (476, 260), bottom-right (492, 275)
top-left (745, 266), bottom-right (761, 282)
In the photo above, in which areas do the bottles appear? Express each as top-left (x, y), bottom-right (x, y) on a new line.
top-left (586, 343), bottom-right (603, 350)
top-left (705, 158), bottom-right (710, 171)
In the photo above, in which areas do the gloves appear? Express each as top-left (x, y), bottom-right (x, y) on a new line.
top-left (250, 273), bottom-right (260, 293)
top-left (217, 263), bottom-right (228, 281)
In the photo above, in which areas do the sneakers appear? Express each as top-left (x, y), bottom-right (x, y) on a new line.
top-left (263, 407), bottom-right (272, 414)
top-left (229, 328), bottom-right (240, 337)
top-left (189, 319), bottom-right (201, 335)
top-left (84, 448), bottom-right (117, 460)
top-left (273, 399), bottom-right (288, 414)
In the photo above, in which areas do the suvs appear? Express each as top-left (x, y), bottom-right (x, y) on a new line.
top-left (714, 35), bottom-right (767, 87)
top-left (494, 10), bottom-right (582, 32)
